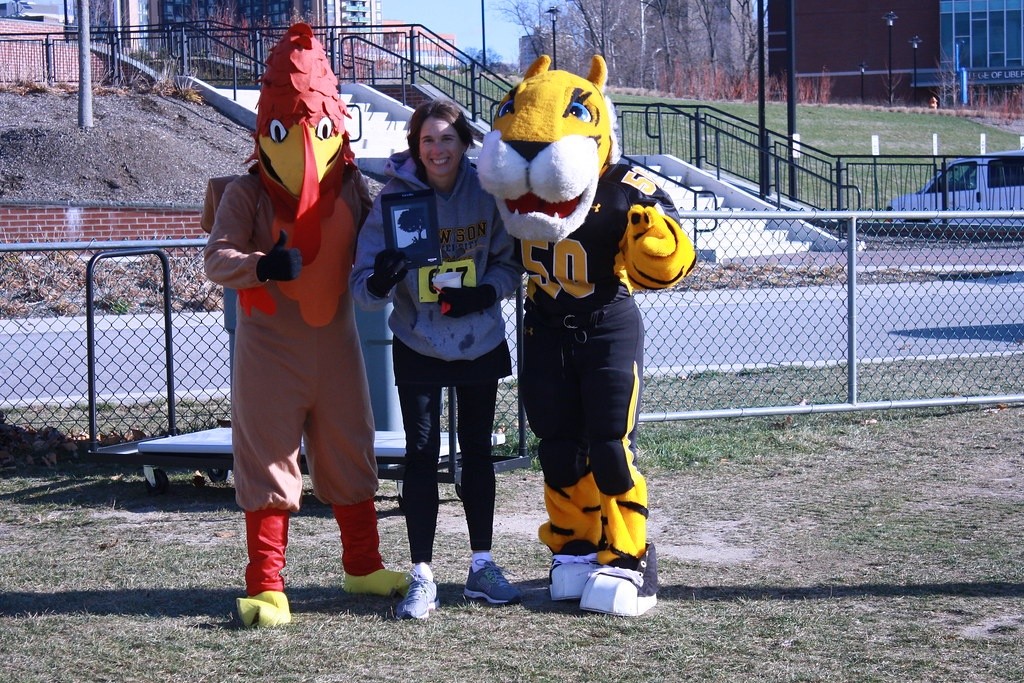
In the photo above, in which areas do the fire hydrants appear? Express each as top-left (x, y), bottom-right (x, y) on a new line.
top-left (929, 96), bottom-right (938, 109)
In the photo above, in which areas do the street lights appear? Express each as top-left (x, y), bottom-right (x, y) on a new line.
top-left (907, 35), bottom-right (922, 107)
top-left (857, 63), bottom-right (869, 104)
top-left (882, 12), bottom-right (898, 107)
top-left (545, 6), bottom-right (562, 70)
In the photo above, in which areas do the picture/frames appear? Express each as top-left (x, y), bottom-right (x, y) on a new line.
top-left (381, 187), bottom-right (443, 271)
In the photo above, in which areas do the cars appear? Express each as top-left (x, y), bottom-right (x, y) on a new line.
top-left (886, 150), bottom-right (1024, 225)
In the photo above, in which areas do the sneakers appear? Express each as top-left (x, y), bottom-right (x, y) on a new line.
top-left (464, 561), bottom-right (520, 605)
top-left (394, 573), bottom-right (439, 620)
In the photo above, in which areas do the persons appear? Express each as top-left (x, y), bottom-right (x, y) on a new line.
top-left (351, 98), bottom-right (526, 619)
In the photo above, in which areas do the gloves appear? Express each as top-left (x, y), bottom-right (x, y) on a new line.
top-left (364, 248), bottom-right (410, 296)
top-left (436, 285), bottom-right (496, 316)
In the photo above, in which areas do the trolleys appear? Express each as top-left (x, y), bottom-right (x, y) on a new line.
top-left (86, 247), bottom-right (531, 511)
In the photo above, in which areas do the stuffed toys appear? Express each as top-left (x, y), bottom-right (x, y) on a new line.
top-left (204, 22), bottom-right (413, 629)
top-left (476, 55), bottom-right (697, 618)
